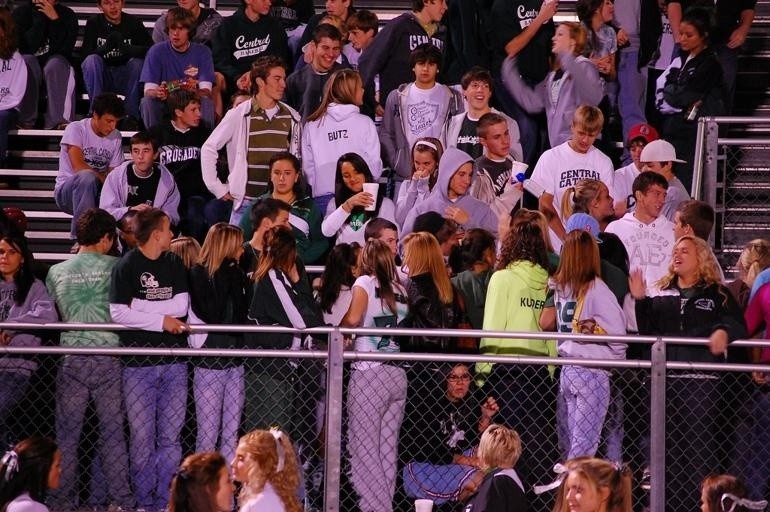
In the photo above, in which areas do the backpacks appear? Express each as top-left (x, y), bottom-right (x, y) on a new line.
top-left (407, 297), bottom-right (449, 351)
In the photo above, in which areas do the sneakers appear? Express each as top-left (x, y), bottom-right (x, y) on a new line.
top-left (44, 117), bottom-right (71, 131)
top-left (15, 121), bottom-right (36, 130)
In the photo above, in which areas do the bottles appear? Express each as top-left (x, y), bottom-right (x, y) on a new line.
top-left (160, 81), bottom-right (168, 100)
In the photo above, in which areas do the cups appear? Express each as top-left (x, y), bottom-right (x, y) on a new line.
top-left (511, 160), bottom-right (529, 185)
top-left (363, 183), bottom-right (379, 211)
top-left (414, 499), bottom-right (434, 512)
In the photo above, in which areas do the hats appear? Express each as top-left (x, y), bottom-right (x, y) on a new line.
top-left (639, 138), bottom-right (687, 165)
top-left (3, 207), bottom-right (28, 231)
top-left (565, 210), bottom-right (603, 245)
top-left (413, 211), bottom-right (447, 238)
top-left (626, 123), bottom-right (660, 147)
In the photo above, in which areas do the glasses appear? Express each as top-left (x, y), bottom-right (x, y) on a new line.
top-left (447, 373), bottom-right (471, 382)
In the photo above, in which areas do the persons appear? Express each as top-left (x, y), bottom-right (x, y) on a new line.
top-left (0, 0), bottom-right (770, 512)
